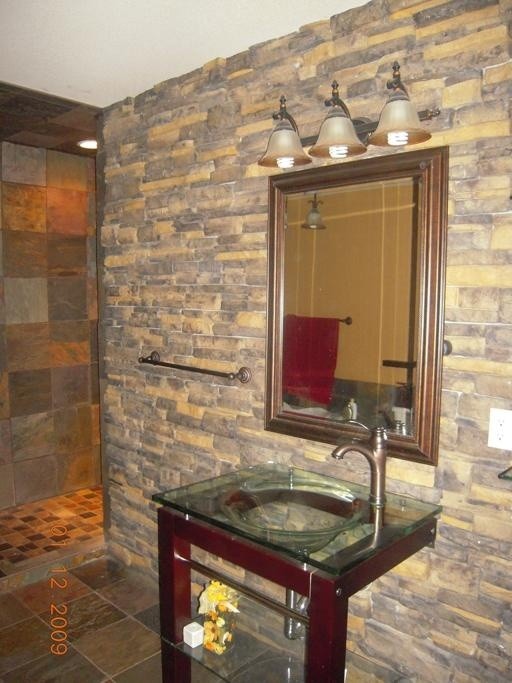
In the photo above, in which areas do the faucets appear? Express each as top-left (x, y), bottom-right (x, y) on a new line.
top-left (330, 424), bottom-right (388, 508)
top-left (331, 504), bottom-right (384, 560)
top-left (342, 418), bottom-right (404, 434)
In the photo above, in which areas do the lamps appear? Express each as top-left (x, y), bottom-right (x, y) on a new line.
top-left (256, 60), bottom-right (432, 170)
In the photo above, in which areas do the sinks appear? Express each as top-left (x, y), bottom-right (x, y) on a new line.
top-left (217, 477), bottom-right (364, 550)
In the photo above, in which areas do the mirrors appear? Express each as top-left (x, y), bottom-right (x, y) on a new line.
top-left (263, 145), bottom-right (449, 469)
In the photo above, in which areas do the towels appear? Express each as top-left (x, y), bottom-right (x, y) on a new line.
top-left (282, 312), bottom-right (341, 408)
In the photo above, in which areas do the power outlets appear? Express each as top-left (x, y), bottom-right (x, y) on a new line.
top-left (486, 407), bottom-right (512, 450)
